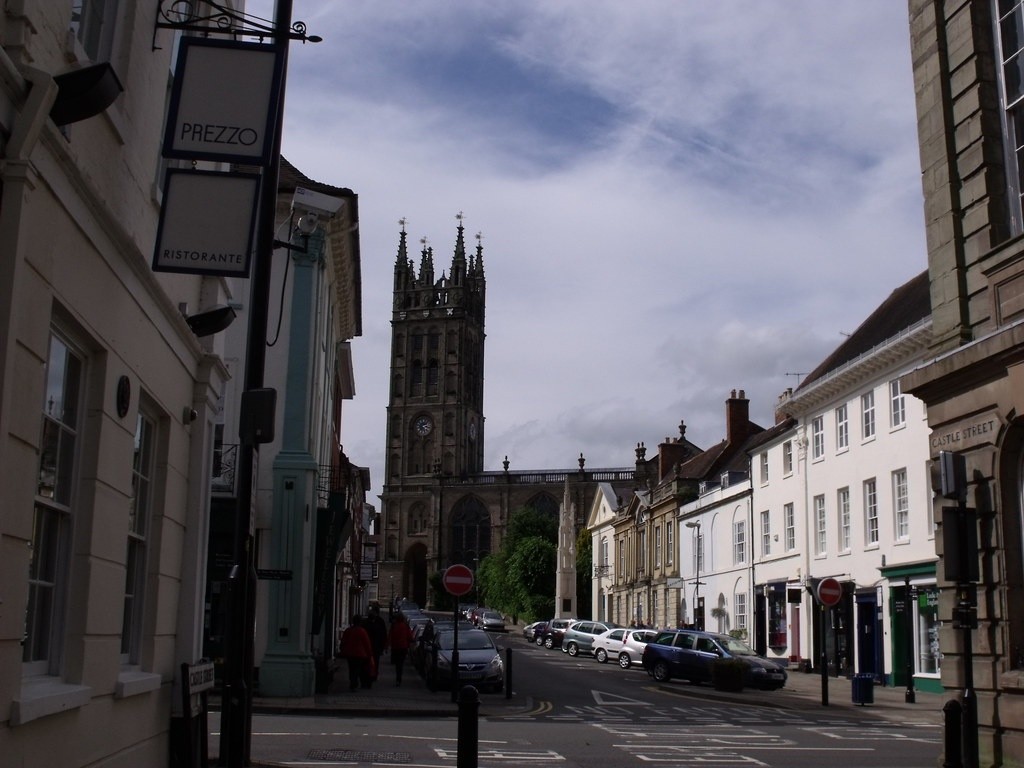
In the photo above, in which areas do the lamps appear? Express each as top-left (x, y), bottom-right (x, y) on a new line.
top-left (187, 303), bottom-right (236, 337)
top-left (49, 61), bottom-right (125, 127)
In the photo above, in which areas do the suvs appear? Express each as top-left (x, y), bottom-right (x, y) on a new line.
top-left (641, 628), bottom-right (789, 692)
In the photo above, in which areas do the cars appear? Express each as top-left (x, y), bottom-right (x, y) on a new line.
top-left (618, 629), bottom-right (659, 669)
top-left (396, 600), bottom-right (507, 694)
top-left (560, 620), bottom-right (619, 657)
top-left (521, 620), bottom-right (549, 643)
top-left (542, 618), bottom-right (579, 650)
top-left (590, 627), bottom-right (639, 664)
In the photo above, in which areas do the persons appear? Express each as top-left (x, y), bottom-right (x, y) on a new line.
top-left (339, 612), bottom-right (390, 692)
top-left (387, 611), bottom-right (415, 687)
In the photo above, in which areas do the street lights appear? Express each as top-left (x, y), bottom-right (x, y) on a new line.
top-left (685, 521), bottom-right (701, 599)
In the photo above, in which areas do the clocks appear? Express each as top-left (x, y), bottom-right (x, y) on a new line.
top-left (469, 423), bottom-right (476, 441)
top-left (413, 416), bottom-right (433, 436)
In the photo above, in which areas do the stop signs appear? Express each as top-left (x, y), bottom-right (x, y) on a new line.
top-left (442, 565), bottom-right (475, 596)
top-left (817, 577), bottom-right (843, 608)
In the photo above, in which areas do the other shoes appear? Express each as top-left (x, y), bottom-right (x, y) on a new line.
top-left (395, 679), bottom-right (402, 688)
